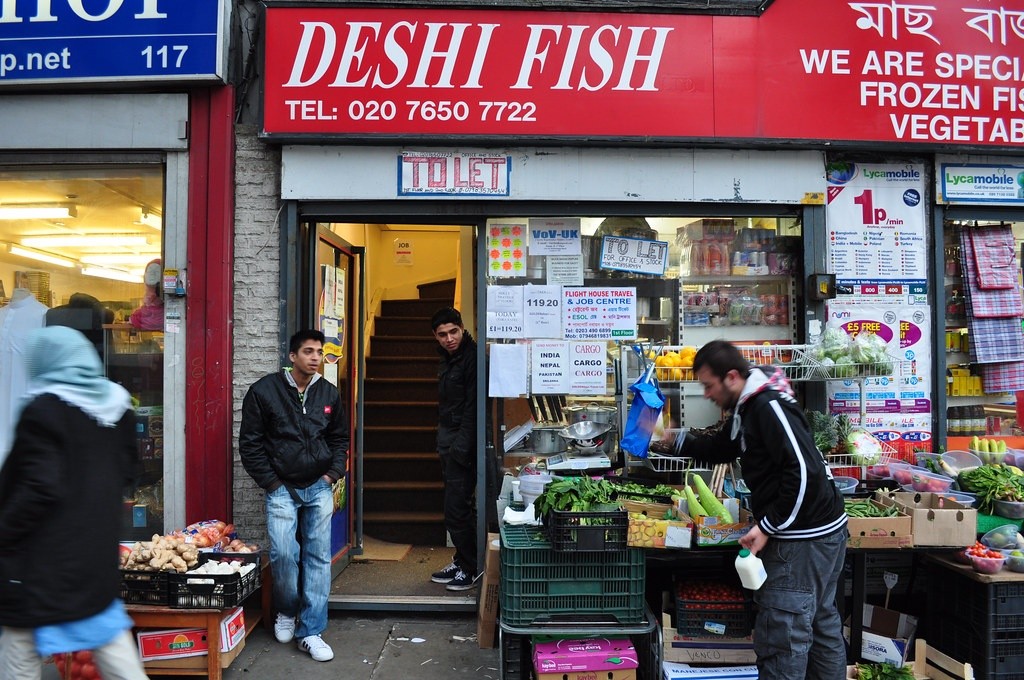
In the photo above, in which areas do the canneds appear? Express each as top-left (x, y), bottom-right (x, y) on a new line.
top-left (683, 291), bottom-right (788, 326)
top-left (730, 251), bottom-right (767, 267)
top-left (733, 228), bottom-right (775, 253)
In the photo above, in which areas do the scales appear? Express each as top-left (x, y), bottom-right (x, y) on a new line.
top-left (545, 449), bottom-right (611, 477)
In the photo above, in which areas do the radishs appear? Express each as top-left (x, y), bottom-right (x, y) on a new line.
top-left (938, 459), bottom-right (978, 477)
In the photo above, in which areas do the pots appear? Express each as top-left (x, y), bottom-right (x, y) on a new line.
top-left (563, 403), bottom-right (617, 452)
top-left (531, 421), bottom-right (567, 453)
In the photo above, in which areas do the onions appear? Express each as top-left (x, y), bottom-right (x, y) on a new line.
top-left (166, 520), bottom-right (259, 607)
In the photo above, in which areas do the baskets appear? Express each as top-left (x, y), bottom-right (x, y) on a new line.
top-left (675, 597), bottom-right (758, 637)
top-left (116, 549), bottom-right (264, 609)
top-left (540, 483), bottom-right (628, 550)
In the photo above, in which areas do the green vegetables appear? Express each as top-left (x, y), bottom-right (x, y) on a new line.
top-left (962, 462), bottom-right (1024, 515)
top-left (853, 662), bottom-right (915, 680)
top-left (533, 474), bottom-right (676, 541)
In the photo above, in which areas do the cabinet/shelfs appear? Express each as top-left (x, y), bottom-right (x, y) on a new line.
top-left (123, 605), bottom-right (262, 680)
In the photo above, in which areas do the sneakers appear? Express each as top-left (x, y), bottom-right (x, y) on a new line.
top-left (446, 567), bottom-right (478, 591)
top-left (298, 634), bottom-right (334, 661)
top-left (275, 612), bottom-right (296, 642)
top-left (431, 563), bottom-right (460, 583)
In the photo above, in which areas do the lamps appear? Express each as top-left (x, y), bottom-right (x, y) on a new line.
top-left (0, 202), bottom-right (161, 284)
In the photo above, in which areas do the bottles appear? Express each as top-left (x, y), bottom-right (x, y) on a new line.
top-left (120, 315), bottom-right (130, 341)
top-left (732, 228), bottom-right (801, 277)
top-left (735, 549), bottom-right (767, 590)
top-left (944, 246), bottom-right (961, 276)
top-left (946, 406), bottom-right (986, 436)
top-left (947, 286), bottom-right (967, 321)
top-left (761, 294), bottom-right (788, 324)
top-left (680, 239), bottom-right (732, 277)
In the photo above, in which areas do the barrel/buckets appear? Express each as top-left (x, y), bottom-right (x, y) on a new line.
top-left (526, 229), bottom-right (657, 280)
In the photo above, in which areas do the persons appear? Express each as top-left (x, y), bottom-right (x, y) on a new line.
top-left (45, 288), bottom-right (114, 463)
top-left (239, 330), bottom-right (348, 662)
top-left (0, 327), bottom-right (151, 680)
top-left (641, 340), bottom-right (848, 680)
top-left (431, 305), bottom-right (492, 590)
top-left (0, 287), bottom-right (51, 468)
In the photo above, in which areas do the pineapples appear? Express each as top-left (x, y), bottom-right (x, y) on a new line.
top-left (820, 413), bottom-right (852, 463)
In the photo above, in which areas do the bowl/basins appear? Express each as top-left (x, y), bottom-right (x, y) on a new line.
top-left (949, 488), bottom-right (1024, 518)
top-left (903, 470), bottom-right (955, 493)
top-left (969, 447), bottom-right (1024, 471)
top-left (914, 451), bottom-right (982, 479)
top-left (862, 457), bottom-right (911, 478)
top-left (519, 475), bottom-right (564, 509)
top-left (935, 493), bottom-right (976, 508)
top-left (558, 420), bottom-right (612, 455)
top-left (833, 476), bottom-right (859, 494)
top-left (955, 547), bottom-right (1024, 573)
top-left (888, 463), bottom-right (931, 486)
top-left (981, 524), bottom-right (1018, 549)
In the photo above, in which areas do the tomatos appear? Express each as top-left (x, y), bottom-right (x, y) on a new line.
top-left (954, 540), bottom-right (1004, 574)
top-left (875, 466), bottom-right (950, 492)
top-left (676, 580), bottom-right (745, 611)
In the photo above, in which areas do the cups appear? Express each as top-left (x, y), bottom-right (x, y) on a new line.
top-left (512, 481), bottom-right (523, 501)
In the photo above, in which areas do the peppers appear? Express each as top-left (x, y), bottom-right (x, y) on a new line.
top-left (844, 496), bottom-right (899, 518)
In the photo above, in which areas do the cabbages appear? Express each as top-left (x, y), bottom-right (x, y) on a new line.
top-left (809, 326), bottom-right (893, 377)
top-left (844, 432), bottom-right (881, 464)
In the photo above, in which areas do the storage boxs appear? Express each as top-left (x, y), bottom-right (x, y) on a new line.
top-left (136, 405), bottom-right (163, 459)
top-left (476, 476), bottom-right (1024, 680)
top-left (119, 548), bottom-right (264, 669)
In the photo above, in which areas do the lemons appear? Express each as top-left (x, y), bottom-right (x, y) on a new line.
top-left (643, 346), bottom-right (697, 380)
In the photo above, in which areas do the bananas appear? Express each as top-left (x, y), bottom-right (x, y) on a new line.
top-left (970, 435), bottom-right (1006, 462)
